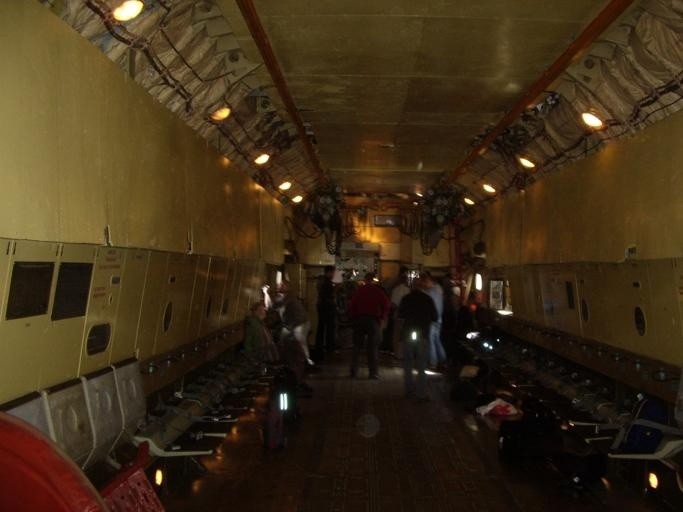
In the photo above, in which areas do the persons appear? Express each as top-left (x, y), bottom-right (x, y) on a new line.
top-left (241, 264), bottom-right (481, 406)
